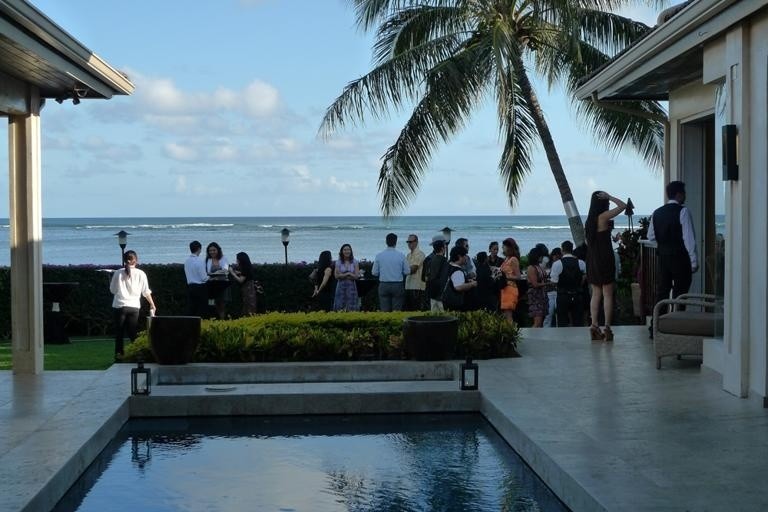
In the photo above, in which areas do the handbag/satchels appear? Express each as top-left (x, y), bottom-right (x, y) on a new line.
top-left (430, 298), bottom-right (445, 312)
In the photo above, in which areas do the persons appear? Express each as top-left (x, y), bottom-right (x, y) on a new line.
top-left (206, 241), bottom-right (233, 320)
top-left (421, 235), bottom-right (621, 328)
top-left (333, 244), bottom-right (359, 310)
top-left (109, 250), bottom-right (156, 362)
top-left (229, 252), bottom-right (257, 317)
top-left (646, 180), bottom-right (699, 338)
top-left (585, 190), bottom-right (628, 341)
top-left (183, 241), bottom-right (209, 317)
top-left (405, 234), bottom-right (425, 312)
top-left (371, 233), bottom-right (411, 311)
top-left (308, 251), bottom-right (332, 313)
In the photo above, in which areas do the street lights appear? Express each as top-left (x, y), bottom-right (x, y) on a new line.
top-left (437, 225), bottom-right (456, 261)
top-left (280, 226), bottom-right (292, 264)
top-left (113, 229), bottom-right (132, 265)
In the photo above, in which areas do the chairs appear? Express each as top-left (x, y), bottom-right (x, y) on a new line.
top-left (649, 288), bottom-right (723, 371)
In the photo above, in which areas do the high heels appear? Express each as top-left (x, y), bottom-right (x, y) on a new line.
top-left (603, 325), bottom-right (613, 341)
top-left (589, 324), bottom-right (605, 340)
